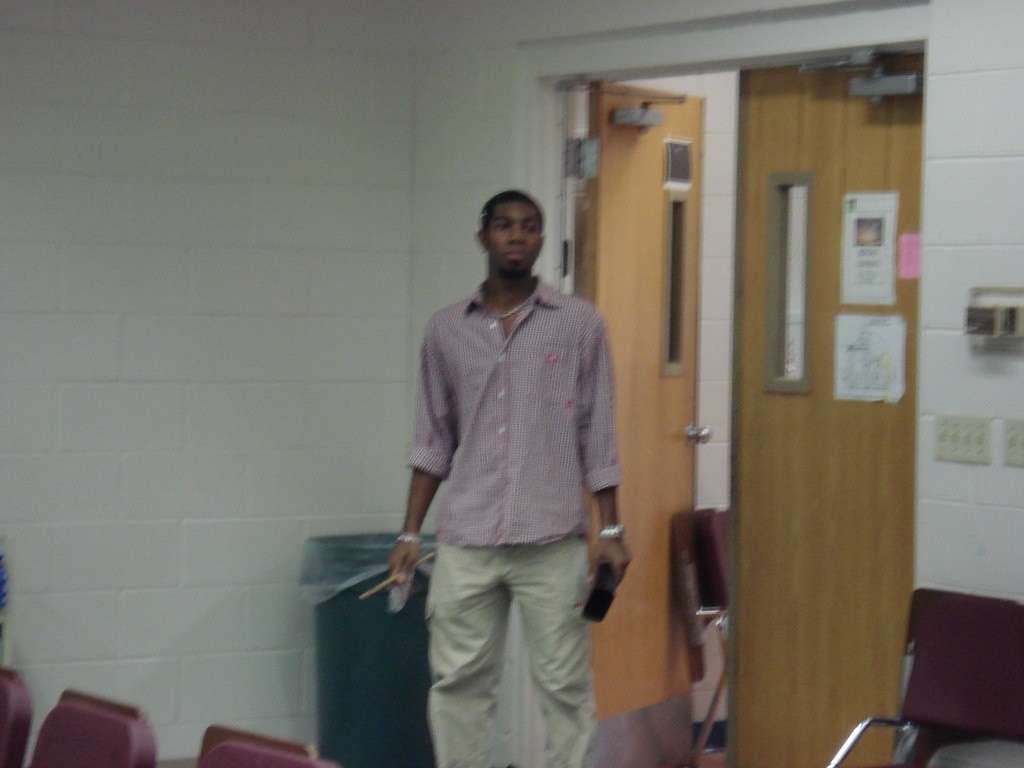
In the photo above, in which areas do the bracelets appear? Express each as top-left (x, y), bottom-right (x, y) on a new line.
top-left (396, 531), bottom-right (422, 543)
top-left (599, 524), bottom-right (624, 539)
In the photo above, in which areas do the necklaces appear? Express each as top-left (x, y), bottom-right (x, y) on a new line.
top-left (491, 296), bottom-right (528, 319)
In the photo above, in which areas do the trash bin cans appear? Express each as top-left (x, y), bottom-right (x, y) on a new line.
top-left (304, 529), bottom-right (443, 768)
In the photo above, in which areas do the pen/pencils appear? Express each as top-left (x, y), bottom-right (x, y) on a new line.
top-left (357, 550), bottom-right (435, 600)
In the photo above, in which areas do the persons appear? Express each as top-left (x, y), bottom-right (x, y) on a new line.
top-left (390, 190), bottom-right (630, 768)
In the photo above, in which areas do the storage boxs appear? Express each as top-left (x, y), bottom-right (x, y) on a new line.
top-left (581, 563), bottom-right (616, 622)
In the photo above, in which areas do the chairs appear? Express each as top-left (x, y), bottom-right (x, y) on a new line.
top-left (827, 587), bottom-right (1024, 768)
top-left (696, 508), bottom-right (731, 757)
top-left (0, 668), bottom-right (340, 768)
